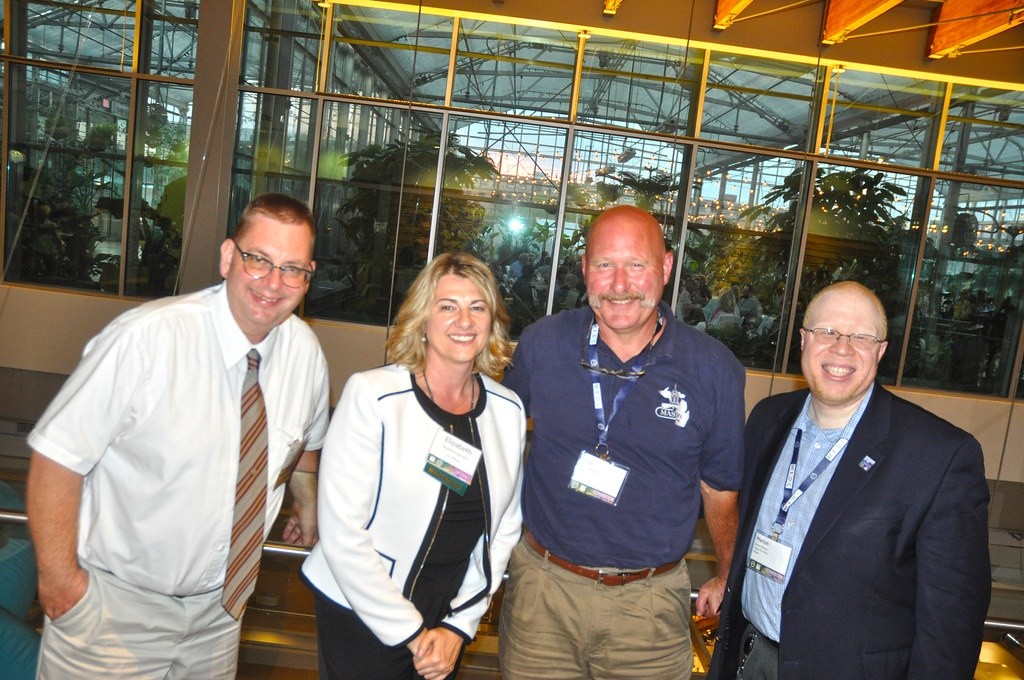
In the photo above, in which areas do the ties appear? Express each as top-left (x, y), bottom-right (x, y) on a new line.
top-left (221, 348), bottom-right (268, 621)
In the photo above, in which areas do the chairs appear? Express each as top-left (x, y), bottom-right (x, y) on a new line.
top-left (753, 316), bottom-right (777, 338)
top-left (503, 263), bottom-right (537, 307)
top-left (716, 314), bottom-right (747, 332)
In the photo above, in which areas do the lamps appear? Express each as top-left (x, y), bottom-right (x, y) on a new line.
top-left (617, 148), bottom-right (636, 163)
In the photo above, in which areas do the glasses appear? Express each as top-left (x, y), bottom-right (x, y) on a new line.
top-left (803, 328), bottom-right (885, 353)
top-left (233, 240), bottom-right (314, 289)
top-left (580, 314), bottom-right (658, 379)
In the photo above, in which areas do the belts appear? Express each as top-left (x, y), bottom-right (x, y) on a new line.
top-left (750, 622), bottom-right (779, 648)
top-left (524, 529), bottom-right (679, 586)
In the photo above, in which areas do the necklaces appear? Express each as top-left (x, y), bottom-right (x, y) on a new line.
top-left (423, 369), bottom-right (474, 412)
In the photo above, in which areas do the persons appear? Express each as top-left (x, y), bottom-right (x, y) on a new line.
top-left (6, 195), bottom-right (65, 284)
top-left (499, 205), bottom-right (746, 680)
top-left (296, 252), bottom-right (525, 680)
top-left (26, 191), bottom-right (331, 680)
top-left (705, 281), bottom-right (993, 680)
top-left (496, 252), bottom-right (763, 361)
